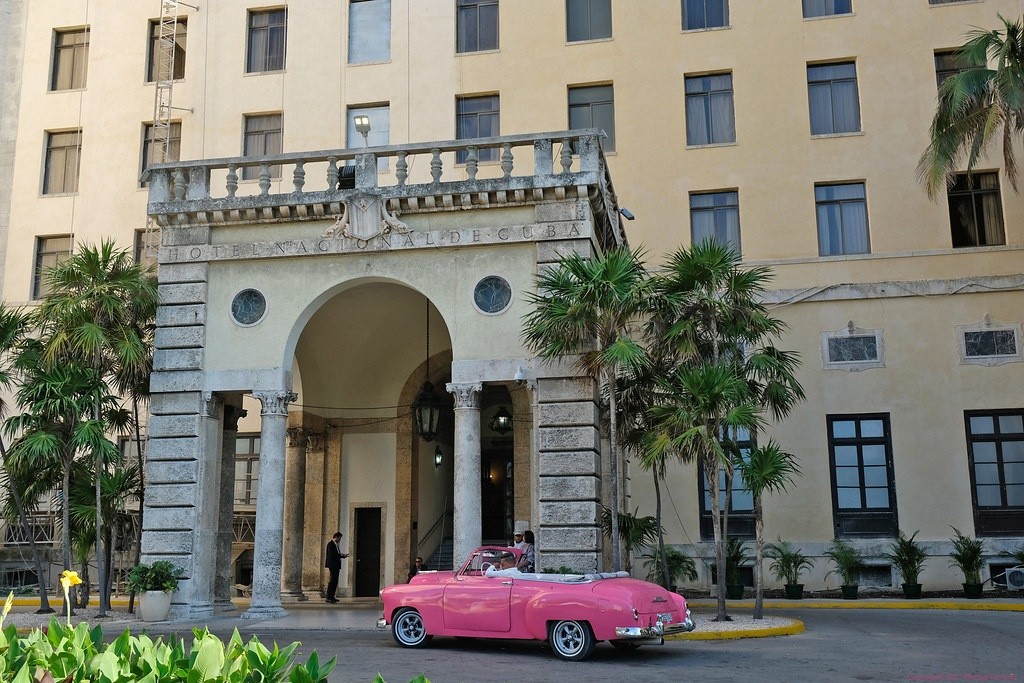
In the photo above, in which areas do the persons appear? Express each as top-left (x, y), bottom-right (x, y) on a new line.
top-left (484, 553), bottom-right (522, 578)
top-left (512, 530), bottom-right (526, 549)
top-left (407, 557), bottom-right (428, 584)
top-left (517, 530), bottom-right (535, 573)
top-left (324, 531), bottom-right (350, 605)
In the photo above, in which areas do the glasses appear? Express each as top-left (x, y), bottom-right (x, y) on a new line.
top-left (415, 561), bottom-right (421, 563)
top-left (515, 534), bottom-right (522, 537)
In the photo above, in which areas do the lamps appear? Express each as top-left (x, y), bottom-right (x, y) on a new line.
top-left (410, 297), bottom-right (442, 443)
top-left (489, 406), bottom-right (514, 434)
top-left (435, 445), bottom-right (443, 468)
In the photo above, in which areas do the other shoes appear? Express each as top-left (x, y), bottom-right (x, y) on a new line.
top-left (325, 597), bottom-right (340, 604)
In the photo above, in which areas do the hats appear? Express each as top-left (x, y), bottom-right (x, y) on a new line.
top-left (513, 530), bottom-right (524, 535)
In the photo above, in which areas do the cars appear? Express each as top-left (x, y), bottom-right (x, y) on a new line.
top-left (374, 545), bottom-right (697, 662)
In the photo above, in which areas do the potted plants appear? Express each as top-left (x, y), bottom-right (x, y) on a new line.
top-left (642, 545), bottom-right (698, 594)
top-left (760, 537), bottom-right (817, 600)
top-left (718, 535), bottom-right (757, 600)
top-left (821, 535), bottom-right (866, 600)
top-left (946, 523), bottom-right (984, 598)
top-left (123, 559), bottom-right (186, 622)
top-left (877, 527), bottom-right (933, 598)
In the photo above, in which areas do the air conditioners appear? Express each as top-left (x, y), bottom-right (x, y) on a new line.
top-left (1005, 568), bottom-right (1024, 591)
